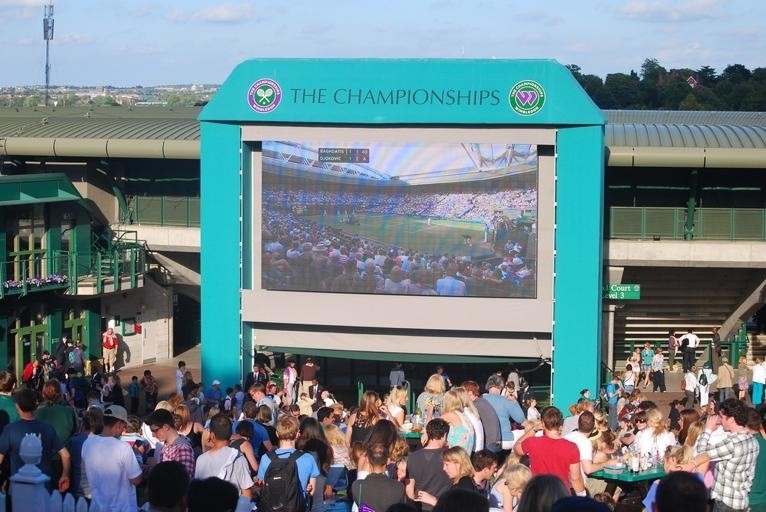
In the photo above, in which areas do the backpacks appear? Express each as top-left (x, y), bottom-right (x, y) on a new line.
top-left (261, 449), bottom-right (307, 512)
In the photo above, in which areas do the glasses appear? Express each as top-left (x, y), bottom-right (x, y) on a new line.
top-left (635, 418), bottom-right (646, 423)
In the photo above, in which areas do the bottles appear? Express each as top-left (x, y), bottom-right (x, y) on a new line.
top-left (411, 401), bottom-right (422, 432)
top-left (630, 441), bottom-right (640, 473)
top-left (651, 436), bottom-right (660, 469)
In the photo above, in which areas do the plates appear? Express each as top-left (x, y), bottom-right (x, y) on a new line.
top-left (602, 464), bottom-right (626, 470)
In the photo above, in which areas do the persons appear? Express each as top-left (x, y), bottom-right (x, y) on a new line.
top-left (1, 328), bottom-right (765, 510)
top-left (261, 187), bottom-right (537, 298)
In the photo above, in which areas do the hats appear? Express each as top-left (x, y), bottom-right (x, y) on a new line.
top-left (102, 404), bottom-right (133, 427)
